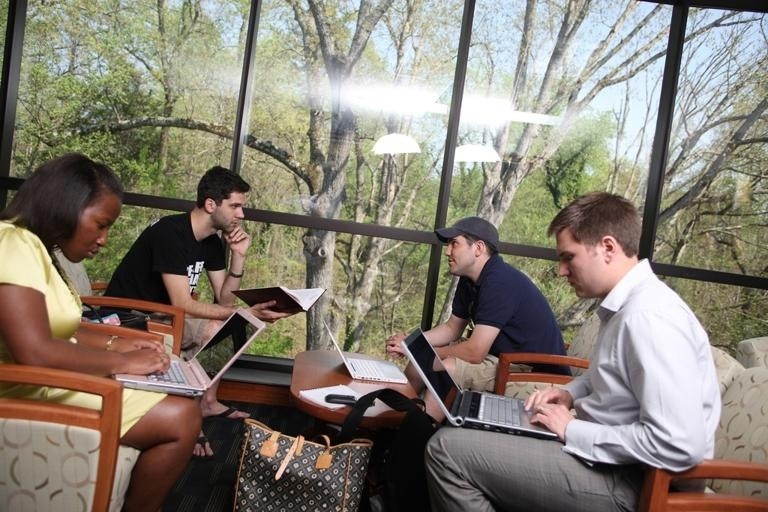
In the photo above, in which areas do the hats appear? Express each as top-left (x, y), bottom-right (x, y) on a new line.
top-left (434, 217), bottom-right (499, 251)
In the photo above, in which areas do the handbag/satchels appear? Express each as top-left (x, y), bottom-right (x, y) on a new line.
top-left (82, 309), bottom-right (149, 331)
top-left (365, 414), bottom-right (440, 512)
top-left (233, 418), bottom-right (372, 512)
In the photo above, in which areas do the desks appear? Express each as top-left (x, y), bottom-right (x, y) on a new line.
top-left (288, 350), bottom-right (420, 439)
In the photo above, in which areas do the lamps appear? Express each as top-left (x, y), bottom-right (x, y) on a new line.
top-left (369, 131), bottom-right (423, 155)
top-left (454, 144), bottom-right (499, 164)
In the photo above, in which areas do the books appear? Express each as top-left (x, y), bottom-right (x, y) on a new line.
top-left (298, 383), bottom-right (364, 412)
top-left (229, 286), bottom-right (327, 314)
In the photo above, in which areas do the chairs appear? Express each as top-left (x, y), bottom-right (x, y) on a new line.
top-left (736, 336), bottom-right (768, 369)
top-left (0, 323), bottom-right (164, 512)
top-left (492, 307), bottom-right (606, 399)
top-left (635, 341), bottom-right (768, 512)
top-left (53, 244), bottom-right (199, 356)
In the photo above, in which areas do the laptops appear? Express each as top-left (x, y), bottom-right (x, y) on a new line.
top-left (322, 319), bottom-right (409, 385)
top-left (402, 326), bottom-right (560, 440)
top-left (113, 306), bottom-right (267, 396)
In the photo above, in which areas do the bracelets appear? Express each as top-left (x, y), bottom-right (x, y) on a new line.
top-left (228, 268), bottom-right (245, 279)
top-left (104, 335), bottom-right (119, 351)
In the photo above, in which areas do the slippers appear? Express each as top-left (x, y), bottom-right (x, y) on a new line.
top-left (192, 436), bottom-right (216, 460)
top-left (205, 405), bottom-right (252, 421)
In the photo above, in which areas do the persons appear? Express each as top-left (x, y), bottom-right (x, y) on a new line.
top-left (1, 149), bottom-right (199, 512)
top-left (420, 190), bottom-right (721, 512)
top-left (382, 217), bottom-right (573, 427)
top-left (99, 166), bottom-right (299, 457)
